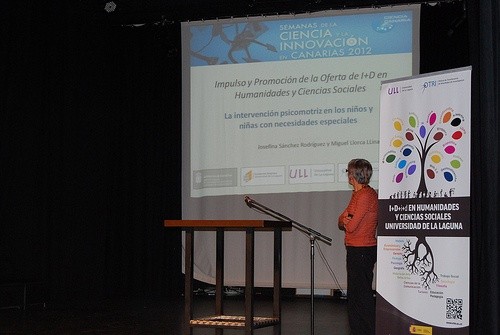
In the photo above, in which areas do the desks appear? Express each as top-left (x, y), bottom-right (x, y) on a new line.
top-left (163, 219), bottom-right (292, 335)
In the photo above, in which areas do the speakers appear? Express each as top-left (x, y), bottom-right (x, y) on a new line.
top-left (0, 281), bottom-right (31, 312)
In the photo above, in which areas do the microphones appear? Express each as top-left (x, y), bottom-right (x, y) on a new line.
top-left (245, 196), bottom-right (252, 203)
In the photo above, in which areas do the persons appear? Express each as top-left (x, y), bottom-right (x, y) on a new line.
top-left (338, 158), bottom-right (379, 335)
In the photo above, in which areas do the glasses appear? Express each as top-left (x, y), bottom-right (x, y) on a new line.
top-left (345, 169), bottom-right (350, 173)
